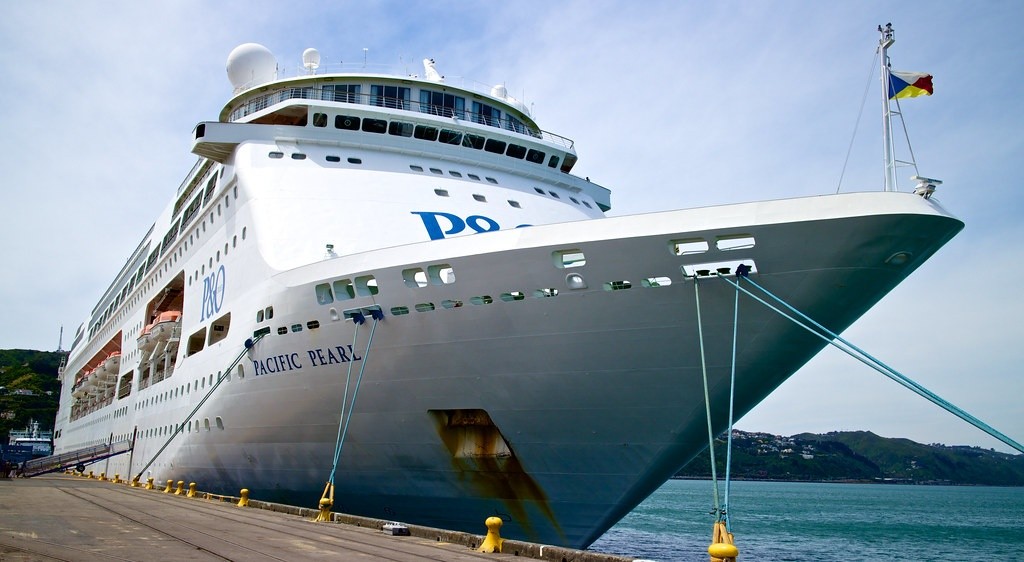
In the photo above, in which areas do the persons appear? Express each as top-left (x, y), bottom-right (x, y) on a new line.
top-left (0, 456), bottom-right (67, 480)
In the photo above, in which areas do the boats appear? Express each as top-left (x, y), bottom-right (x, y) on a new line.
top-left (52, 23), bottom-right (966, 551)
top-left (70, 311), bottom-right (185, 417)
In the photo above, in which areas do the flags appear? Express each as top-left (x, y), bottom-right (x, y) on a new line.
top-left (888, 69), bottom-right (935, 101)
